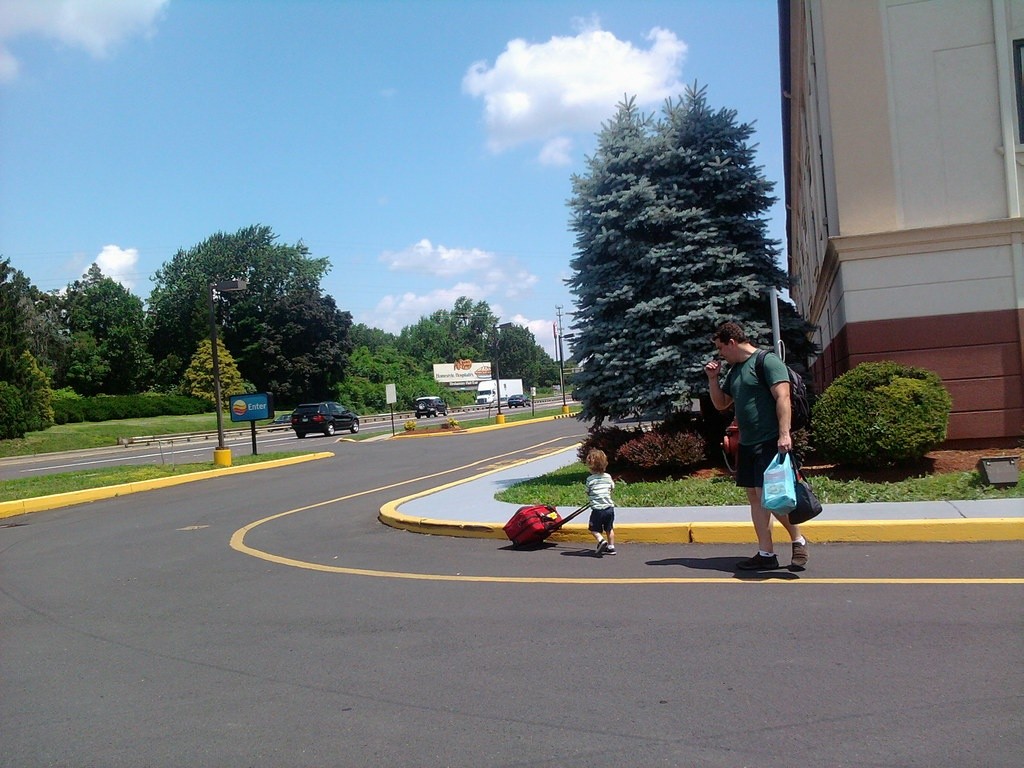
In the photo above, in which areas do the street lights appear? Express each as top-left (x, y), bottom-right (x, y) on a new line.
top-left (206, 276), bottom-right (247, 449)
top-left (492, 322), bottom-right (514, 415)
top-left (558, 333), bottom-right (575, 406)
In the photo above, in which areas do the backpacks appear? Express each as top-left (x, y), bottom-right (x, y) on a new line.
top-left (727, 349), bottom-right (811, 432)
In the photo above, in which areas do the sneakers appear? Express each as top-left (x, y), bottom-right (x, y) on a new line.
top-left (594, 539), bottom-right (608, 554)
top-left (601, 547), bottom-right (617, 555)
top-left (735, 554), bottom-right (780, 570)
top-left (790, 534), bottom-right (809, 571)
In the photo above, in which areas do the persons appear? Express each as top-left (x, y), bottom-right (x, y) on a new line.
top-left (706, 323), bottom-right (808, 571)
top-left (585, 450), bottom-right (616, 554)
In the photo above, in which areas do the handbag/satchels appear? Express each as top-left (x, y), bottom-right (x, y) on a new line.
top-left (780, 449), bottom-right (822, 525)
top-left (760, 451), bottom-right (798, 514)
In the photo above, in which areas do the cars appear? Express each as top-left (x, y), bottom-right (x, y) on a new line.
top-left (267, 414), bottom-right (291, 432)
top-left (507, 394), bottom-right (531, 409)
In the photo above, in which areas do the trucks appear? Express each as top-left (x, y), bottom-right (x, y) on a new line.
top-left (474, 379), bottom-right (523, 404)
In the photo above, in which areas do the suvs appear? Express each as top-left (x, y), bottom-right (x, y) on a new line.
top-left (415, 397), bottom-right (448, 419)
top-left (292, 402), bottom-right (360, 438)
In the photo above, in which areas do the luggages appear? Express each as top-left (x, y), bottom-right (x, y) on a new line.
top-left (502, 501), bottom-right (592, 550)
